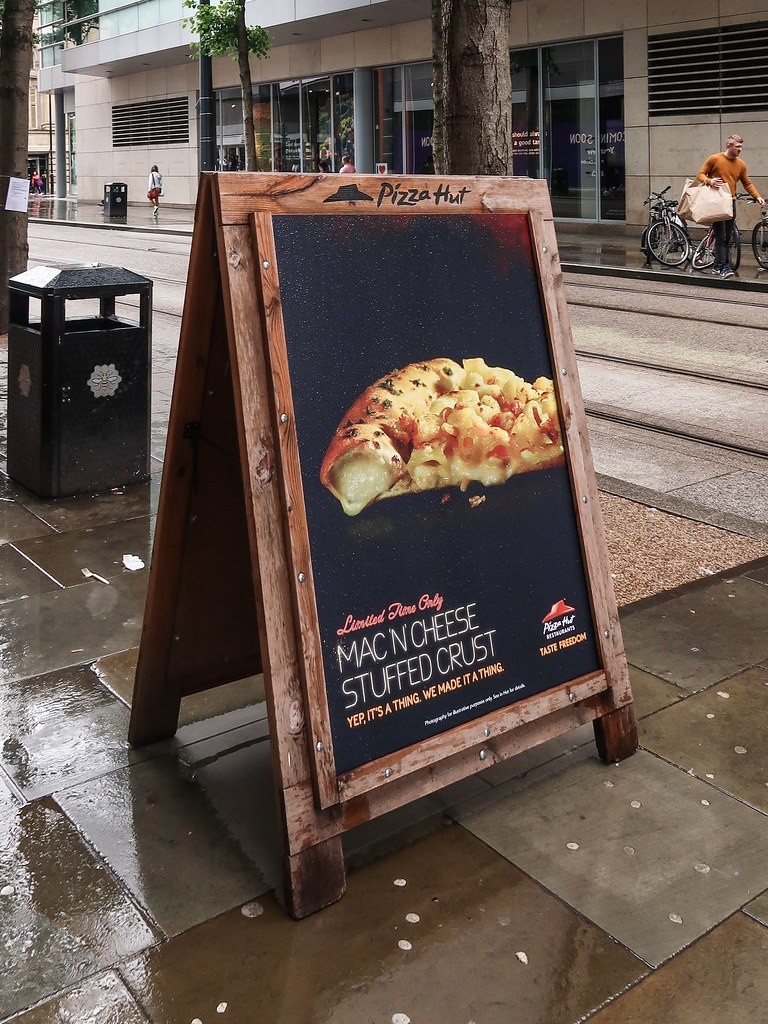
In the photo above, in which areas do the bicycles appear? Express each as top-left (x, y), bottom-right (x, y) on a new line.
top-left (640, 186), bottom-right (768, 272)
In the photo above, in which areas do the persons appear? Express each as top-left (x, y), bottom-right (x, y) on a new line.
top-left (27, 169), bottom-right (47, 193)
top-left (148, 165), bottom-right (161, 213)
top-left (696, 136), bottom-right (765, 279)
top-left (220, 155), bottom-right (354, 173)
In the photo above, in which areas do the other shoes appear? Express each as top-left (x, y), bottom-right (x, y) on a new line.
top-left (154, 206), bottom-right (159, 215)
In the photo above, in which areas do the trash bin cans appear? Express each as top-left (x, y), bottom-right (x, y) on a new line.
top-left (6, 258), bottom-right (155, 504)
top-left (103, 181), bottom-right (128, 224)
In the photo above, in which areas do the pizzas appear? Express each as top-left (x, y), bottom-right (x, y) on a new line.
top-left (320, 357), bottom-right (569, 517)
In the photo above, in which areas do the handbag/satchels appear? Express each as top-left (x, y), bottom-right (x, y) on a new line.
top-left (675, 178), bottom-right (712, 226)
top-left (687, 179), bottom-right (733, 224)
top-left (36, 179), bottom-right (43, 187)
top-left (670, 215), bottom-right (688, 244)
top-left (147, 188), bottom-right (158, 198)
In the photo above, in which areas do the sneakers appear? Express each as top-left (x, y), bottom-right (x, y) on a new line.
top-left (720, 265), bottom-right (735, 280)
top-left (712, 266), bottom-right (733, 275)
